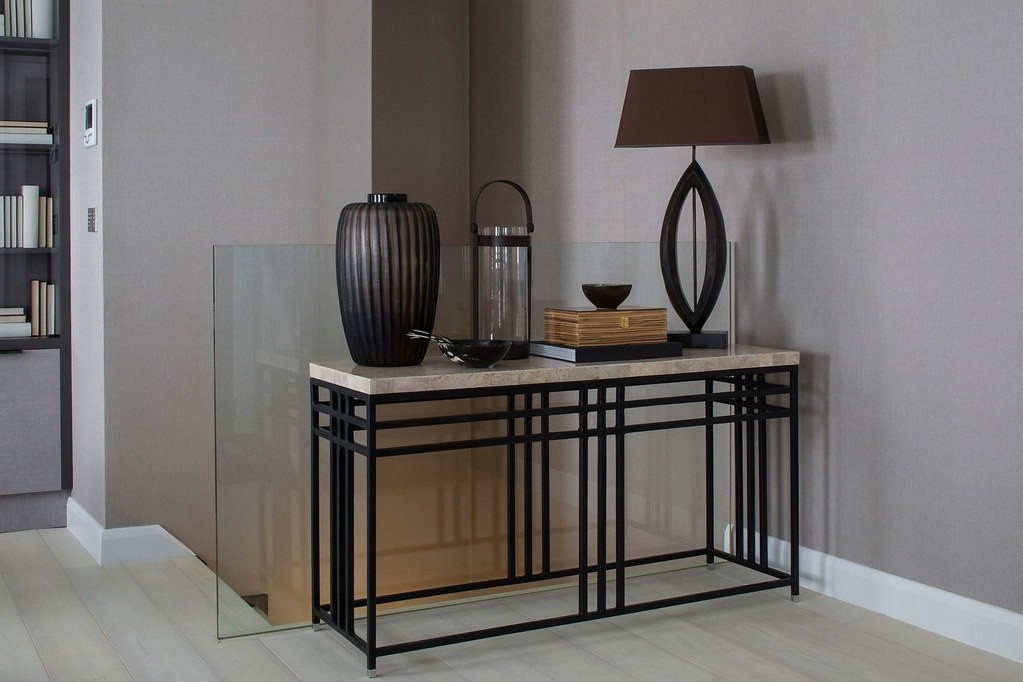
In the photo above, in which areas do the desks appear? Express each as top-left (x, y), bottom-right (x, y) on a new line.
top-left (309, 345), bottom-right (801, 680)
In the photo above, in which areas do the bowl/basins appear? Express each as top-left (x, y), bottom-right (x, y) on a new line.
top-left (440, 339), bottom-right (512, 368)
top-left (582, 284), bottom-right (632, 309)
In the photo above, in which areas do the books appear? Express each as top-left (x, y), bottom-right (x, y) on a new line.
top-left (0, 185), bottom-right (53, 249)
top-left (0, 0), bottom-right (54, 39)
top-left (0, 120), bottom-right (54, 146)
top-left (0, 279), bottom-right (56, 339)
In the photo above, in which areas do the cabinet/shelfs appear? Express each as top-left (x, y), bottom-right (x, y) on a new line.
top-left (0, 0), bottom-right (74, 496)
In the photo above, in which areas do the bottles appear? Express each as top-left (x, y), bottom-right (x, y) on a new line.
top-left (336, 193), bottom-right (440, 367)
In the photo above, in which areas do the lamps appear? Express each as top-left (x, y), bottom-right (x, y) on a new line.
top-left (611, 64), bottom-right (771, 348)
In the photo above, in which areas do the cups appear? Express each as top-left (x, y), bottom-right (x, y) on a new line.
top-left (478, 227), bottom-right (530, 347)
